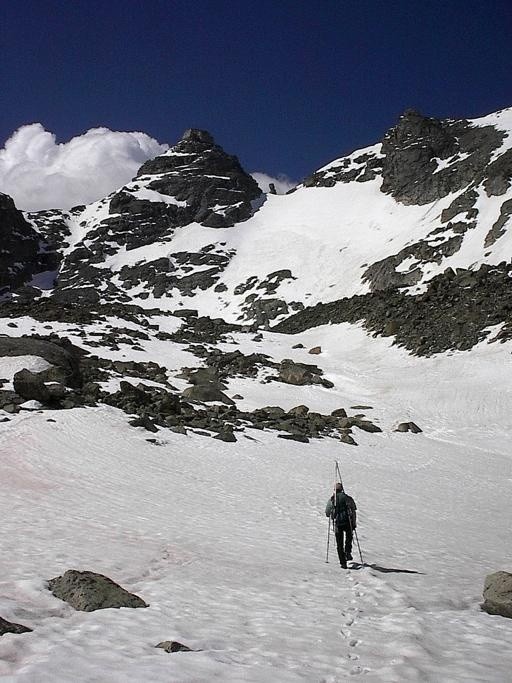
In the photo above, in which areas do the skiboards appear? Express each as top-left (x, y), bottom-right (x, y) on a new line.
top-left (332, 459), bottom-right (339, 534)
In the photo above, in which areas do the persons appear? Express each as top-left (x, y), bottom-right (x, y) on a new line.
top-left (324, 483), bottom-right (357, 569)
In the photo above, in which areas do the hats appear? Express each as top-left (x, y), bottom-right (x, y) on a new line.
top-left (334, 483), bottom-right (343, 489)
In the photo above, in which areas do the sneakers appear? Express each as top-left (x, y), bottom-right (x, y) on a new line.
top-left (346, 552), bottom-right (353, 560)
top-left (341, 564), bottom-right (348, 569)
